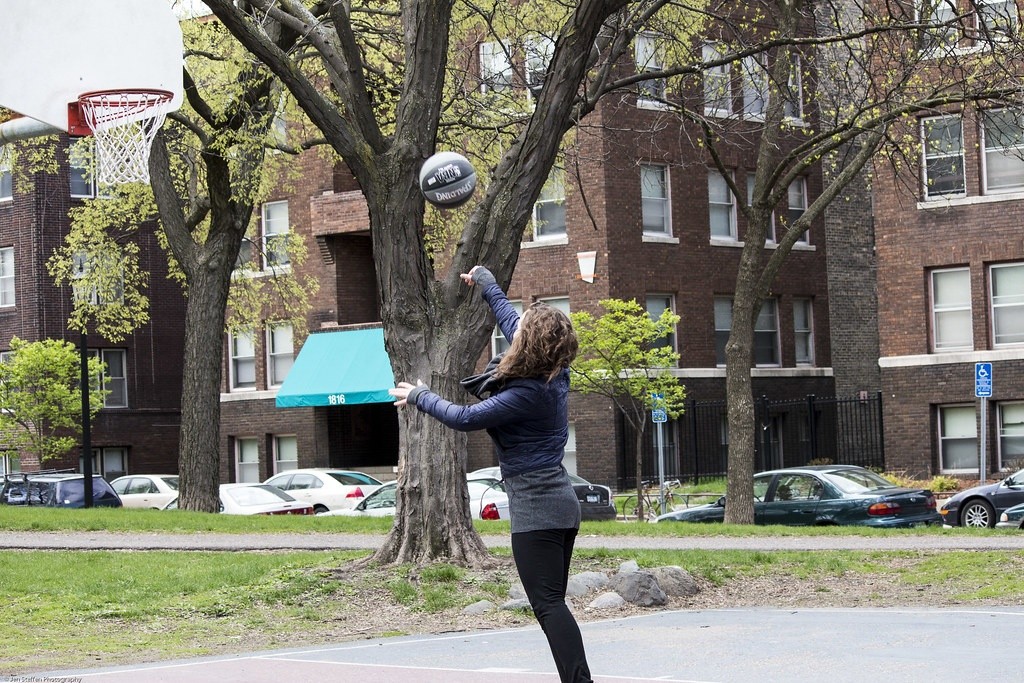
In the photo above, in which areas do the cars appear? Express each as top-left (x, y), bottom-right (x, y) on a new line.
top-left (217, 483), bottom-right (316, 516)
top-left (264, 468), bottom-right (383, 518)
top-left (316, 473), bottom-right (512, 522)
top-left (939, 467), bottom-right (1024, 529)
top-left (470, 465), bottom-right (618, 523)
top-left (653, 464), bottom-right (945, 532)
top-left (109, 474), bottom-right (180, 510)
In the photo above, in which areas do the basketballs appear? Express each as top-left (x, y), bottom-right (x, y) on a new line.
top-left (419, 152), bottom-right (476, 209)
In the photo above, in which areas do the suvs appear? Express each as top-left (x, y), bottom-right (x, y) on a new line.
top-left (0, 471), bottom-right (123, 510)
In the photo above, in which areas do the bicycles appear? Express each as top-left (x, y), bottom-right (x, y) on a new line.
top-left (622, 479), bottom-right (689, 523)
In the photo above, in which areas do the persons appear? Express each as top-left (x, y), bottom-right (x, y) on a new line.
top-left (388, 266), bottom-right (594, 683)
